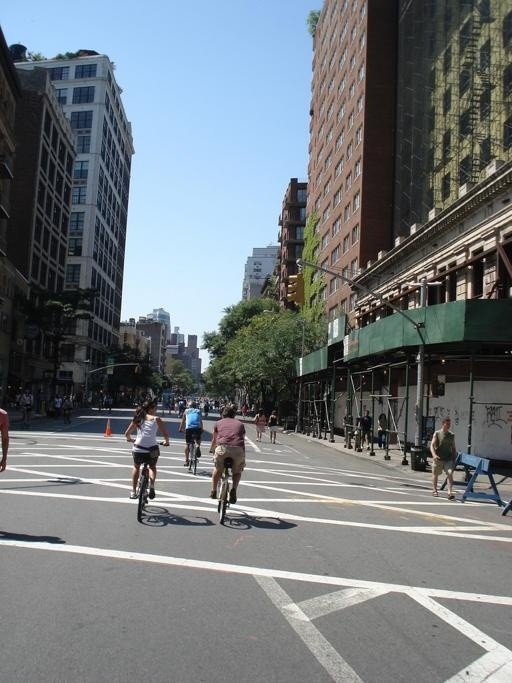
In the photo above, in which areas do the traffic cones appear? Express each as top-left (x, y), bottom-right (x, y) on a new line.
top-left (104, 418), bottom-right (112, 437)
top-left (159, 409), bottom-right (165, 417)
top-left (108, 405), bottom-right (112, 414)
top-left (168, 409), bottom-right (172, 415)
top-left (103, 418), bottom-right (113, 437)
top-left (99, 400), bottom-right (102, 413)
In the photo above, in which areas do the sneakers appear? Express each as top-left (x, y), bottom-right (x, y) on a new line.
top-left (184, 461), bottom-right (189, 466)
top-left (229, 495), bottom-right (236, 502)
top-left (130, 491), bottom-right (137, 498)
top-left (149, 491), bottom-right (155, 497)
top-left (196, 452), bottom-right (201, 456)
top-left (211, 490), bottom-right (217, 498)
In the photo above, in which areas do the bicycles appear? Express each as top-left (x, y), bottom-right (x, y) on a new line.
top-left (128, 441), bottom-right (169, 522)
top-left (62, 407), bottom-right (73, 424)
top-left (180, 430), bottom-right (204, 474)
top-left (179, 406), bottom-right (186, 416)
top-left (19, 406), bottom-right (34, 430)
top-left (210, 449), bottom-right (244, 524)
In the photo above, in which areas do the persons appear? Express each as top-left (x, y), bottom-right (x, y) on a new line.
top-left (254, 408), bottom-right (266, 442)
top-left (378, 413), bottom-right (387, 451)
top-left (358, 410), bottom-right (372, 450)
top-left (171, 393), bottom-right (202, 417)
top-left (430, 418), bottom-right (456, 500)
top-left (343, 409), bottom-right (354, 442)
top-left (13, 390), bottom-right (115, 426)
top-left (209, 406), bottom-right (246, 504)
top-left (204, 398), bottom-right (236, 416)
top-left (0, 408), bottom-right (9, 472)
top-left (267, 410), bottom-right (279, 444)
top-left (125, 400), bottom-right (169, 499)
top-left (241, 405), bottom-right (247, 417)
top-left (178, 401), bottom-right (203, 466)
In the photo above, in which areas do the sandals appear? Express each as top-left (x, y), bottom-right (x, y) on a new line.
top-left (432, 492), bottom-right (438, 497)
top-left (447, 493), bottom-right (455, 500)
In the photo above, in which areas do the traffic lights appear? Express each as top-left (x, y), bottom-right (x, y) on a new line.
top-left (287, 273), bottom-right (304, 305)
top-left (135, 365), bottom-right (142, 373)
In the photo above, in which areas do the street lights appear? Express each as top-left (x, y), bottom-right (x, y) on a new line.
top-left (84, 360), bottom-right (143, 392)
top-left (294, 257), bottom-right (428, 469)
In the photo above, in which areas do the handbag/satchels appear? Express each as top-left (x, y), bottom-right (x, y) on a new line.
top-left (268, 415), bottom-right (274, 427)
top-left (133, 444), bottom-right (160, 459)
top-left (255, 414), bottom-right (259, 424)
